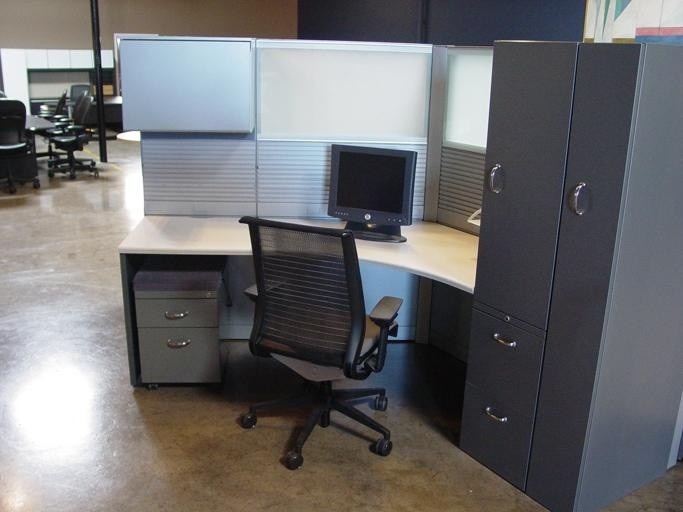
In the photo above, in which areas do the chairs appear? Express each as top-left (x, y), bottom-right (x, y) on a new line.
top-left (0, 83), bottom-right (97, 193)
top-left (239, 216), bottom-right (402, 470)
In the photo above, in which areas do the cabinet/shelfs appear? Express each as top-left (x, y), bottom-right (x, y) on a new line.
top-left (460, 42), bottom-right (682, 511)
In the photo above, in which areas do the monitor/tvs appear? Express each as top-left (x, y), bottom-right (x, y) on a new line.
top-left (326, 144), bottom-right (417, 243)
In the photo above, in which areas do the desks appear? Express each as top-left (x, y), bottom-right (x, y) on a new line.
top-left (118, 215), bottom-right (478, 387)
